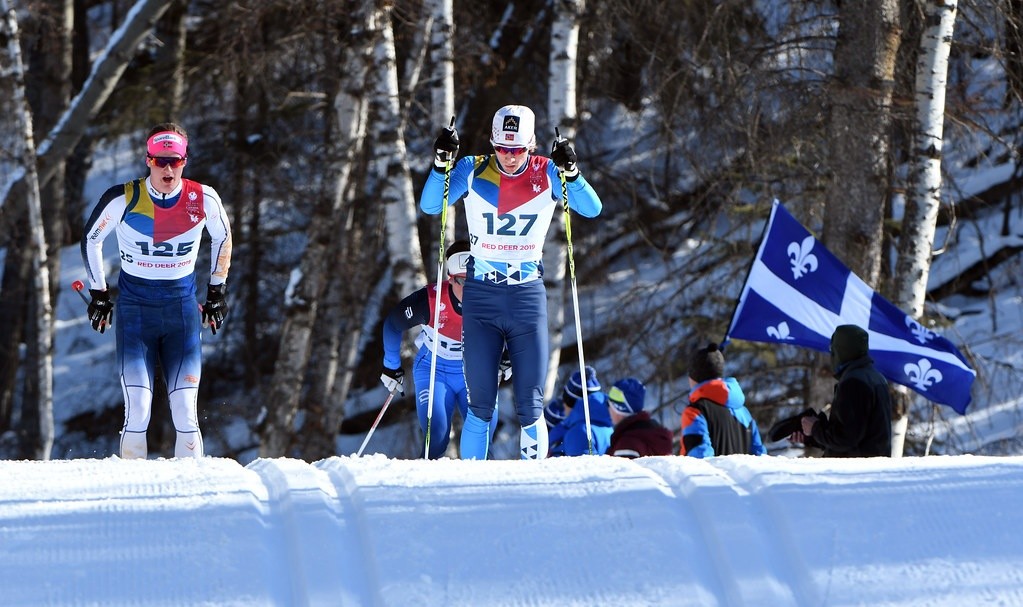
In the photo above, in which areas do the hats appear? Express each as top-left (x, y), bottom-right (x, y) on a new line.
top-left (608, 378), bottom-right (646, 416)
top-left (147, 131), bottom-right (189, 157)
top-left (447, 251), bottom-right (473, 275)
top-left (830, 325), bottom-right (874, 372)
top-left (563, 365), bottom-right (601, 409)
top-left (489, 104), bottom-right (537, 148)
top-left (543, 399), bottom-right (565, 434)
top-left (688, 343), bottom-right (723, 384)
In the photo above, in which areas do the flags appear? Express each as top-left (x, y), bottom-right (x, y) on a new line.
top-left (728, 198), bottom-right (977, 416)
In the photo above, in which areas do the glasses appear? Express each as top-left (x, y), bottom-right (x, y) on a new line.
top-left (449, 276), bottom-right (466, 287)
top-left (146, 157), bottom-right (185, 169)
top-left (495, 146), bottom-right (527, 156)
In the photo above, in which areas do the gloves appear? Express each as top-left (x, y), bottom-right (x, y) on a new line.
top-left (201, 283), bottom-right (229, 335)
top-left (379, 366), bottom-right (405, 397)
top-left (499, 353), bottom-right (513, 380)
top-left (434, 128), bottom-right (460, 173)
top-left (87, 283), bottom-right (115, 335)
top-left (550, 137), bottom-right (579, 183)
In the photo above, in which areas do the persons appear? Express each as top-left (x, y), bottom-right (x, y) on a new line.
top-left (786, 324), bottom-right (891, 458)
top-left (542, 365), bottom-right (673, 461)
top-left (679, 342), bottom-right (766, 459)
top-left (80, 123), bottom-right (233, 459)
top-left (419, 105), bottom-right (604, 460)
top-left (379, 241), bottom-right (513, 460)
